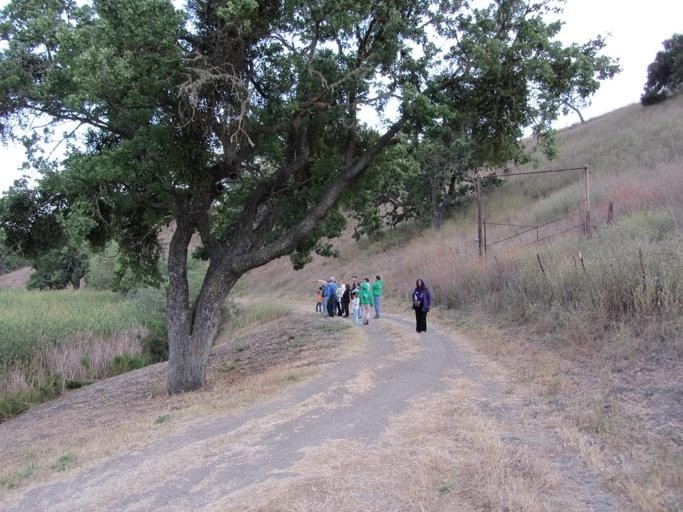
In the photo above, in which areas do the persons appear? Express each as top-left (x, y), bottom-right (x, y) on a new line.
top-left (412, 279), bottom-right (432, 334)
top-left (314, 275), bottom-right (384, 325)
